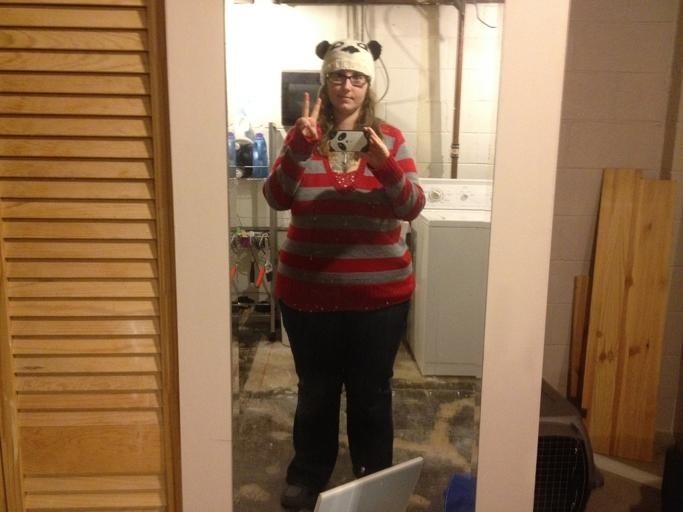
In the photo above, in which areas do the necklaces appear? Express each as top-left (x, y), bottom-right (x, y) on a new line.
top-left (341, 151), bottom-right (347, 163)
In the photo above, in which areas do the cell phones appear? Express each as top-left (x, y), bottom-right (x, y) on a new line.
top-left (329, 131), bottom-right (371, 153)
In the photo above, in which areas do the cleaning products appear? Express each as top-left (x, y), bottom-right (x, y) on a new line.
top-left (228, 130), bottom-right (237, 178)
top-left (251, 132), bottom-right (267, 177)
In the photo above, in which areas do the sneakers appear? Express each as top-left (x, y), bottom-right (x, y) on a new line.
top-left (280, 485), bottom-right (312, 507)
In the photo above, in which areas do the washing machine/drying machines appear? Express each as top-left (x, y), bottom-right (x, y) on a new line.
top-left (407, 176), bottom-right (492, 376)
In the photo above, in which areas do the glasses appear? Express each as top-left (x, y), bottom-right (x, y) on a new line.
top-left (326, 72), bottom-right (370, 86)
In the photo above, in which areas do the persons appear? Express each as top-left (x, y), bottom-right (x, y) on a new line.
top-left (262, 40), bottom-right (424, 509)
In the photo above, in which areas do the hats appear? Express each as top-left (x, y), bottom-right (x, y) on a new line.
top-left (316, 40), bottom-right (381, 86)
